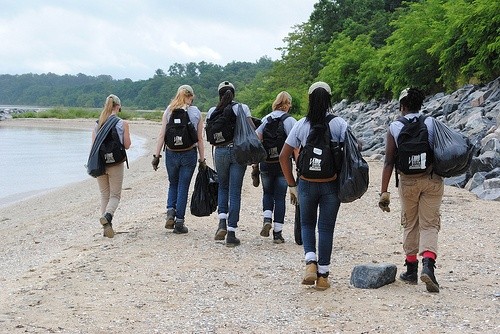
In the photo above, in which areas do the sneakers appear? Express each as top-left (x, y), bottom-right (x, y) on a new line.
top-left (302, 263), bottom-right (330, 290)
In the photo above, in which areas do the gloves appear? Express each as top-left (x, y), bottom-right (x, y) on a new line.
top-left (198, 158), bottom-right (207, 172)
top-left (379, 192), bottom-right (391, 212)
top-left (288, 185), bottom-right (299, 206)
top-left (152, 155), bottom-right (162, 171)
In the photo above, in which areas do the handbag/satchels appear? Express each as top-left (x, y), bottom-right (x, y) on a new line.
top-left (294, 202), bottom-right (303, 245)
top-left (87, 145), bottom-right (104, 177)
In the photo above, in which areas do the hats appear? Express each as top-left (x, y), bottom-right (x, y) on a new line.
top-left (399, 88), bottom-right (410, 111)
top-left (177, 85), bottom-right (194, 102)
top-left (218, 81), bottom-right (235, 101)
top-left (308, 81), bottom-right (331, 97)
top-left (107, 95), bottom-right (121, 112)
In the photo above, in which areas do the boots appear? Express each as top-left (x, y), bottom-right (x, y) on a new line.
top-left (400, 258), bottom-right (440, 293)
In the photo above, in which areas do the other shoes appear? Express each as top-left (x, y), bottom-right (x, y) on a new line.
top-left (164, 209), bottom-right (188, 233)
top-left (259, 218), bottom-right (285, 243)
top-left (252, 165), bottom-right (260, 186)
top-left (214, 223), bottom-right (240, 246)
top-left (100, 213), bottom-right (114, 238)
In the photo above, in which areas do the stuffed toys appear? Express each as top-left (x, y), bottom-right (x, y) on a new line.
top-left (378, 192), bottom-right (391, 212)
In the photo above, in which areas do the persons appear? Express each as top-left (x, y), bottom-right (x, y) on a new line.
top-left (86, 93), bottom-right (131, 238)
top-left (380, 87), bottom-right (444, 292)
top-left (279, 82), bottom-right (362, 291)
top-left (151, 85), bottom-right (209, 233)
top-left (205, 81), bottom-right (256, 246)
top-left (254, 91), bottom-right (300, 243)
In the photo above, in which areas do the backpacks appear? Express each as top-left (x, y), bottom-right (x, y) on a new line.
top-left (96, 120), bottom-right (129, 169)
top-left (205, 102), bottom-right (238, 146)
top-left (262, 113), bottom-right (291, 161)
top-left (163, 108), bottom-right (198, 151)
top-left (395, 115), bottom-right (434, 187)
top-left (296, 114), bottom-right (341, 179)
